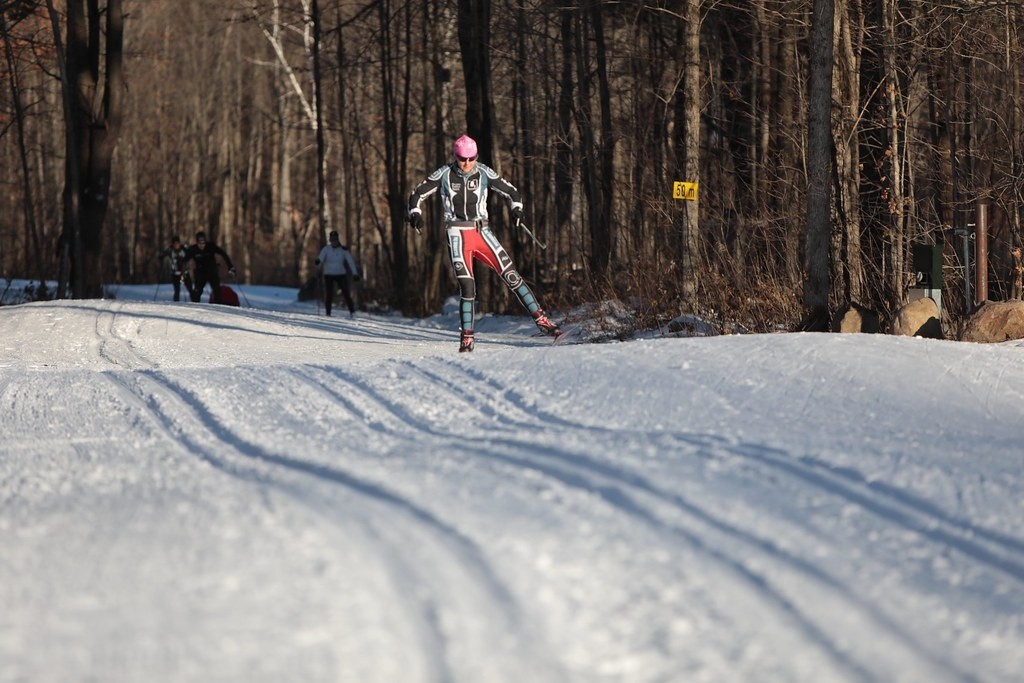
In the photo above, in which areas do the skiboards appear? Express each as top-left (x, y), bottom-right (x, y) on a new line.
top-left (552, 319), bottom-right (584, 345)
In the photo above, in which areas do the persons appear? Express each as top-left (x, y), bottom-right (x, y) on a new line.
top-left (155, 231), bottom-right (237, 304)
top-left (404, 134), bottom-right (560, 353)
top-left (313, 231), bottom-right (361, 318)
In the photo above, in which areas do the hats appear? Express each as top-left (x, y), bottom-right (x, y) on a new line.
top-left (171, 236), bottom-right (181, 243)
top-left (453, 134), bottom-right (478, 158)
top-left (330, 231), bottom-right (338, 241)
top-left (196, 232), bottom-right (205, 241)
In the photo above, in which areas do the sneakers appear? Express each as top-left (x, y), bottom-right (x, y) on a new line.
top-left (533, 312), bottom-right (561, 336)
top-left (459, 327), bottom-right (475, 353)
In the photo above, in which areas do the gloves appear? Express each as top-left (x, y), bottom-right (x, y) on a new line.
top-left (229, 268), bottom-right (234, 273)
top-left (354, 275), bottom-right (360, 281)
top-left (315, 258), bottom-right (320, 265)
top-left (409, 213), bottom-right (424, 229)
top-left (512, 210), bottom-right (525, 225)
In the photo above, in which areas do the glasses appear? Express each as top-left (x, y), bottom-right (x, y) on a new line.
top-left (456, 156), bottom-right (476, 162)
